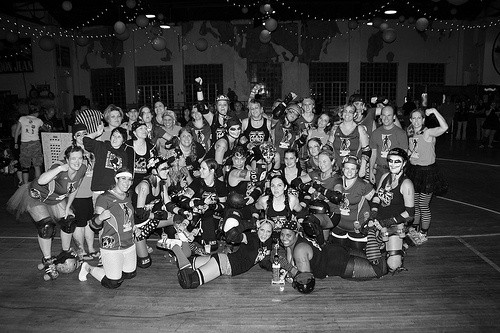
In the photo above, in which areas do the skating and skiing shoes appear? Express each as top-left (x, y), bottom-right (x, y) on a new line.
top-left (38, 256), bottom-right (60, 281)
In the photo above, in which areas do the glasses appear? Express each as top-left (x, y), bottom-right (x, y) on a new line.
top-left (159, 166), bottom-right (170, 172)
top-left (387, 159), bottom-right (403, 163)
top-left (230, 126), bottom-right (241, 131)
top-left (263, 153), bottom-right (274, 156)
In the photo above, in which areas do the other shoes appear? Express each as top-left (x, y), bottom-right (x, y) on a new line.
top-left (89, 250), bottom-right (100, 258)
top-left (76, 251), bottom-right (93, 260)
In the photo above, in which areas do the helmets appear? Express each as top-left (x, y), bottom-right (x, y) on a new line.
top-left (259, 142), bottom-right (277, 153)
top-left (309, 200), bottom-right (330, 214)
top-left (341, 155), bottom-right (362, 167)
top-left (226, 191), bottom-right (247, 209)
top-left (227, 117), bottom-right (242, 130)
top-left (386, 148), bottom-right (408, 164)
top-left (286, 103), bottom-right (302, 118)
top-left (215, 94), bottom-right (229, 101)
top-left (53, 252), bottom-right (77, 274)
top-left (145, 157), bottom-right (168, 173)
top-left (292, 272), bottom-right (315, 294)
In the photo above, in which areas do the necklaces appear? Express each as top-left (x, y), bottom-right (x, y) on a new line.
top-left (112, 187), bottom-right (126, 196)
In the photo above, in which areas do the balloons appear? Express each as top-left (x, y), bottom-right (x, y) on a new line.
top-left (0, 0), bottom-right (430, 52)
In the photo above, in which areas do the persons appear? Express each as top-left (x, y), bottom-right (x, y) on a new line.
top-left (0, 84), bottom-right (499, 258)
top-left (156, 218), bottom-right (278, 289)
top-left (370, 146), bottom-right (416, 273)
top-left (68, 122), bottom-right (102, 261)
top-left (277, 214), bottom-right (397, 280)
top-left (131, 156), bottom-right (193, 270)
top-left (20, 145), bottom-right (88, 280)
top-left (78, 168), bottom-right (138, 289)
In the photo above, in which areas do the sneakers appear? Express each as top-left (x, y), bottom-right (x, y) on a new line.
top-left (156, 238), bottom-right (182, 250)
